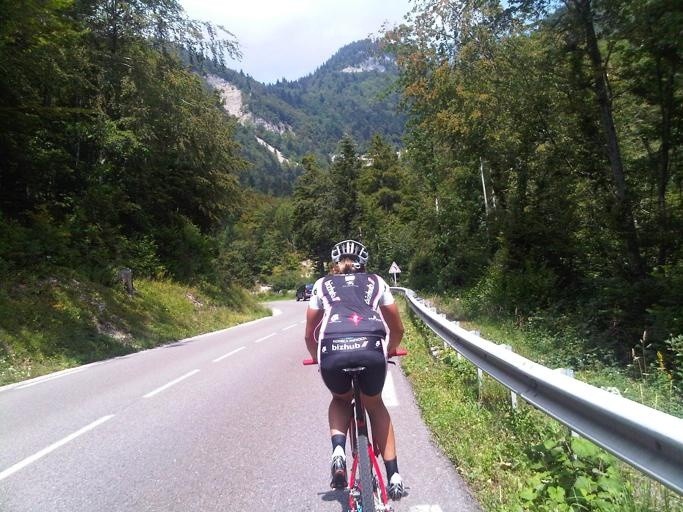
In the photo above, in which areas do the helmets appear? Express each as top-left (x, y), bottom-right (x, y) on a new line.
top-left (330, 239), bottom-right (369, 269)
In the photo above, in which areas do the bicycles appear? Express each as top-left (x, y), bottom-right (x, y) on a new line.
top-left (302, 348), bottom-right (410, 511)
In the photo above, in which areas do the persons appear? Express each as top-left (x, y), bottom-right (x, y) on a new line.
top-left (303, 238), bottom-right (404, 499)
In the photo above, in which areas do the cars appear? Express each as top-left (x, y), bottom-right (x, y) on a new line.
top-left (294, 282), bottom-right (313, 302)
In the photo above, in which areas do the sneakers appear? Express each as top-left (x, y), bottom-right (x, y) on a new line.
top-left (387, 480), bottom-right (404, 502)
top-left (330, 455), bottom-right (349, 489)
